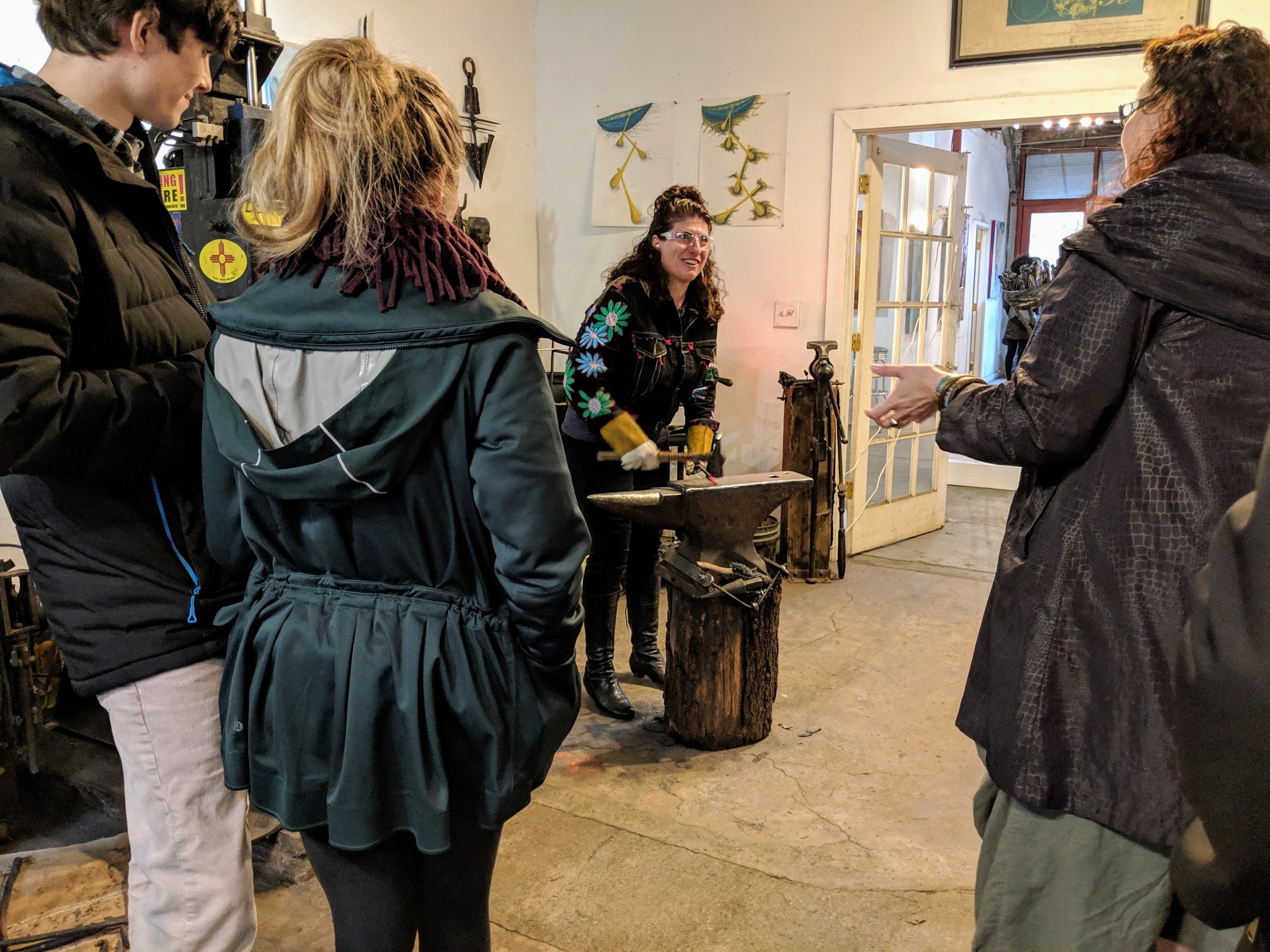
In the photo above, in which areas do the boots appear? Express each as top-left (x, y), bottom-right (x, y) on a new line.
top-left (581, 590), bottom-right (636, 718)
top-left (619, 574), bottom-right (666, 684)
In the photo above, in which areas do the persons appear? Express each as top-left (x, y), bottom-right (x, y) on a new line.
top-left (561, 186), bottom-right (722, 721)
top-left (1170, 431), bottom-right (1270, 930)
top-left (864, 23), bottom-right (1270, 952)
top-left (0, 0), bottom-right (259, 952)
top-left (205, 37), bottom-right (590, 952)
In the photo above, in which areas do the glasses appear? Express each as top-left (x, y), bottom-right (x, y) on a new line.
top-left (1118, 88), bottom-right (1165, 129)
top-left (656, 230), bottom-right (714, 251)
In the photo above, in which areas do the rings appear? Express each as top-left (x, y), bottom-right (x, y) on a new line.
top-left (889, 415), bottom-right (900, 427)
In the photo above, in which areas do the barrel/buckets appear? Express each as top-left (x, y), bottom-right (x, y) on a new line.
top-left (754, 515), bottom-right (780, 562)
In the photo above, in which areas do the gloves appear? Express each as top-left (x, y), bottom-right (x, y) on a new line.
top-left (684, 425), bottom-right (714, 475)
top-left (600, 413), bottom-right (660, 471)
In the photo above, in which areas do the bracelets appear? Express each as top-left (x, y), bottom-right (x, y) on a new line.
top-left (935, 374), bottom-right (959, 396)
